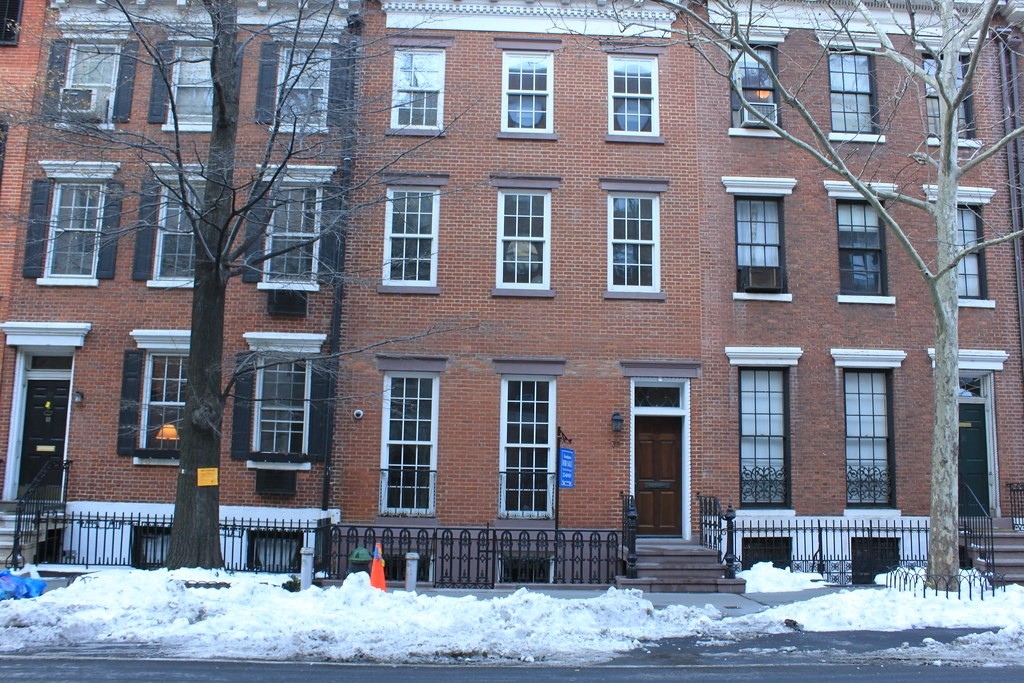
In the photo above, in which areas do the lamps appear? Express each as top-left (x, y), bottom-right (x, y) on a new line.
top-left (74, 391), bottom-right (84, 404)
top-left (611, 412), bottom-right (625, 433)
top-left (155, 425), bottom-right (181, 448)
top-left (757, 90), bottom-right (770, 99)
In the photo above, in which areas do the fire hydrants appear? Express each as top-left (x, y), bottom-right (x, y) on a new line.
top-left (348, 547), bottom-right (372, 576)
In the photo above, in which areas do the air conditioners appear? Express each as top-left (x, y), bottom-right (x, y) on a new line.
top-left (57, 87), bottom-right (109, 124)
top-left (741, 265), bottom-right (783, 293)
top-left (739, 102), bottom-right (778, 129)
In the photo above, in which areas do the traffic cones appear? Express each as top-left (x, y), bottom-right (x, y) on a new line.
top-left (370, 542), bottom-right (386, 592)
